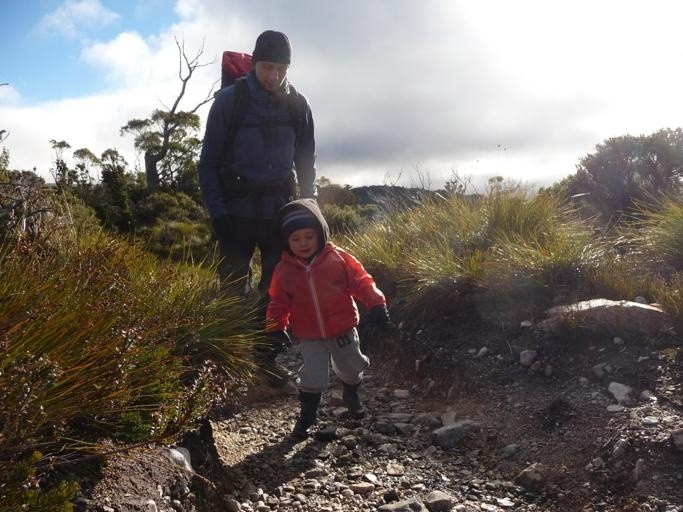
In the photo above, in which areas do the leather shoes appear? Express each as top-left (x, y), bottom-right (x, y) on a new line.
top-left (211, 213), bottom-right (234, 241)
top-left (264, 331), bottom-right (293, 353)
top-left (371, 304), bottom-right (394, 338)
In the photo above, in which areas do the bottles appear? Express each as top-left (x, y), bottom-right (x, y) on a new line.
top-left (252, 29), bottom-right (292, 65)
top-left (281, 206), bottom-right (320, 244)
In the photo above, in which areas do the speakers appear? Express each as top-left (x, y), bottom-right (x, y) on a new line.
top-left (293, 389), bottom-right (323, 439)
top-left (340, 380), bottom-right (366, 417)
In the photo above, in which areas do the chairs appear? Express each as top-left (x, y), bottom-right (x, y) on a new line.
top-left (256, 359), bottom-right (295, 386)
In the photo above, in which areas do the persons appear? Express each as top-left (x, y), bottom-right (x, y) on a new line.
top-left (265, 198), bottom-right (392, 442)
top-left (197, 29), bottom-right (320, 385)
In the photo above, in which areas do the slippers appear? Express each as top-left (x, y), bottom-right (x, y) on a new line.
top-left (220, 50), bottom-right (303, 182)
